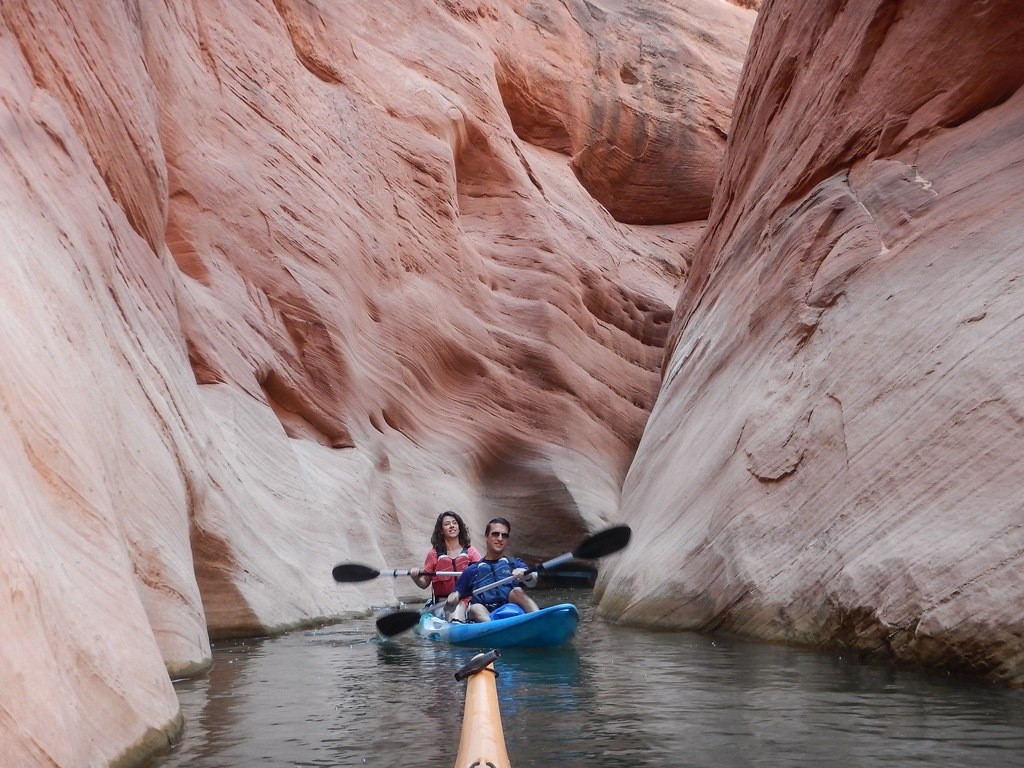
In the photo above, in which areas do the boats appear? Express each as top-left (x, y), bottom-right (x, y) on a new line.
top-left (414, 602), bottom-right (580, 649)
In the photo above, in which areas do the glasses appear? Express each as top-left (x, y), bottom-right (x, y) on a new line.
top-left (488, 531), bottom-right (510, 539)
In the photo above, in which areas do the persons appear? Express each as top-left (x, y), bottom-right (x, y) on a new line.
top-left (410, 511), bottom-right (481, 619)
top-left (444, 518), bottom-right (538, 623)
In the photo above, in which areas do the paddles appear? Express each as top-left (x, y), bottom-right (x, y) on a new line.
top-left (332, 562), bottom-right (598, 590)
top-left (376, 524), bottom-right (631, 639)
